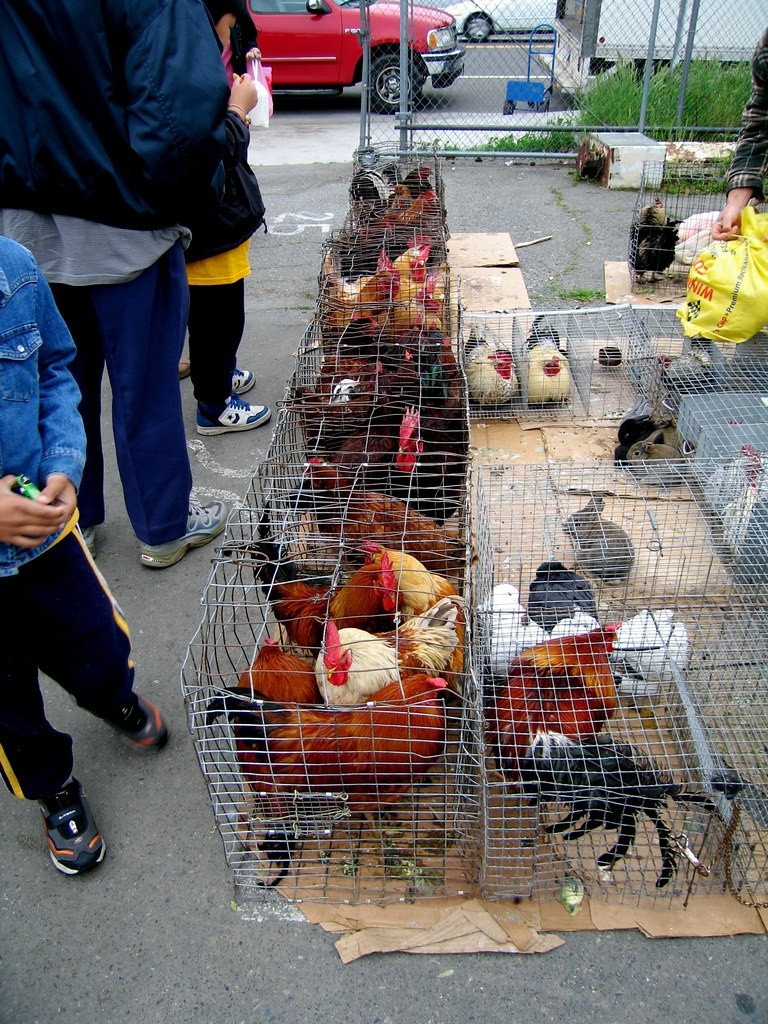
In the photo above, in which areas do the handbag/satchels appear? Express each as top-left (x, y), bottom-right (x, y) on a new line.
top-left (246, 47), bottom-right (274, 117)
top-left (247, 57), bottom-right (270, 129)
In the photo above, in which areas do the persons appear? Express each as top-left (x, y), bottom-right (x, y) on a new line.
top-left (0, 235), bottom-right (170, 876)
top-left (712, 16), bottom-right (768, 241)
top-left (0, 0), bottom-right (272, 571)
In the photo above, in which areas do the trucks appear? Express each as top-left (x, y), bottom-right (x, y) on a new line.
top-left (537, 0), bottom-right (768, 93)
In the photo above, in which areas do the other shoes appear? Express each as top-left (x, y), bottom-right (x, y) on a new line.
top-left (179, 355), bottom-right (191, 379)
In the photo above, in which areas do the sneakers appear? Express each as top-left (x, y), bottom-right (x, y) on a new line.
top-left (78, 525), bottom-right (96, 557)
top-left (140, 499), bottom-right (228, 567)
top-left (104, 691), bottom-right (172, 753)
top-left (197, 392), bottom-right (272, 436)
top-left (38, 776), bottom-right (106, 875)
top-left (232, 368), bottom-right (255, 394)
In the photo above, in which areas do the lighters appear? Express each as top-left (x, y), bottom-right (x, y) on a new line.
top-left (15, 473), bottom-right (41, 501)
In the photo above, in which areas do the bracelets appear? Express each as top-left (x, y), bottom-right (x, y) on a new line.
top-left (228, 104), bottom-right (251, 127)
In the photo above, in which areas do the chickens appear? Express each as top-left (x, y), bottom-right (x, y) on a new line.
top-left (520, 314), bottom-right (570, 406)
top-left (654, 352), bottom-right (768, 558)
top-left (204, 163), bottom-right (478, 869)
top-left (490, 622), bottom-right (712, 891)
top-left (629, 197), bottom-right (684, 283)
top-left (464, 326), bottom-right (519, 406)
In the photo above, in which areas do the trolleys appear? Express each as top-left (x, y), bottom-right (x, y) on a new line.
top-left (503, 24), bottom-right (558, 115)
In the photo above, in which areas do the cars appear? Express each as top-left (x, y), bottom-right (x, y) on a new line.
top-left (437, 0), bottom-right (556, 43)
top-left (244, 0), bottom-right (466, 114)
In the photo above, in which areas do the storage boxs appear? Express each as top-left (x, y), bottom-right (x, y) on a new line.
top-left (678, 392), bottom-right (768, 583)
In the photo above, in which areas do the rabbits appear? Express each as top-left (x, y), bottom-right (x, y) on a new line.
top-left (480, 562), bottom-right (689, 698)
top-left (614, 414), bottom-right (684, 487)
top-left (562, 495), bottom-right (635, 584)
